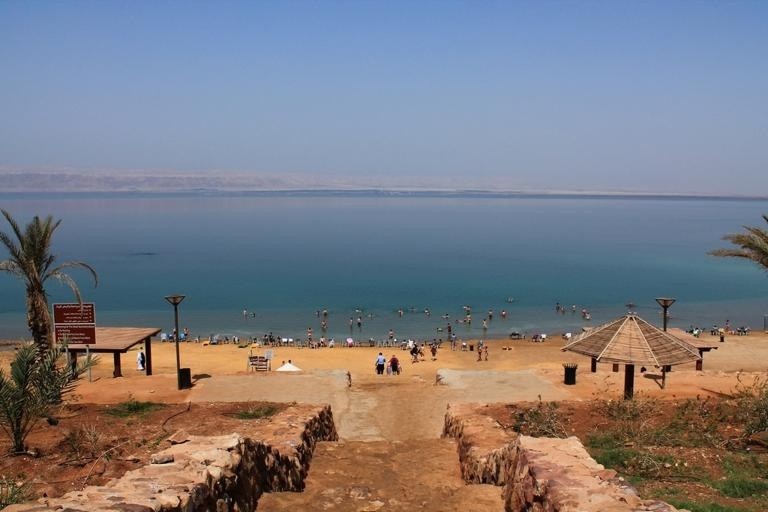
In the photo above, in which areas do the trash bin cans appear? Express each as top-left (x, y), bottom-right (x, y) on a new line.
top-left (179, 368), bottom-right (191, 389)
top-left (470, 344), bottom-right (474, 351)
top-left (564, 363), bottom-right (577, 384)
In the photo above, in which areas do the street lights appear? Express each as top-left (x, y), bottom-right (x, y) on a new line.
top-left (654, 297), bottom-right (676, 389)
top-left (164, 293), bottom-right (185, 390)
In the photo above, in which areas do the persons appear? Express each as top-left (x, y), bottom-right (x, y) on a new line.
top-left (137, 346), bottom-right (145, 371)
top-left (685, 319), bottom-right (752, 342)
